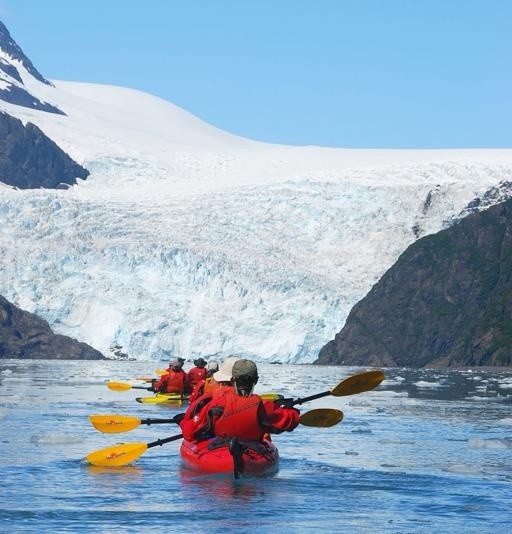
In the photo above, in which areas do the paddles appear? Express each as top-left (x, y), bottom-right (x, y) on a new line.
top-left (86, 369), bottom-right (384, 467)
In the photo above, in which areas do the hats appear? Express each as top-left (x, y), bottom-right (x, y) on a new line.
top-left (211, 356), bottom-right (240, 383)
top-left (193, 357), bottom-right (208, 367)
top-left (208, 360), bottom-right (220, 372)
top-left (168, 356), bottom-right (187, 370)
top-left (231, 358), bottom-right (258, 384)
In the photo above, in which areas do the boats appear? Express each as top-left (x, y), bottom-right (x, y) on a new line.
top-left (171, 435), bottom-right (280, 473)
top-left (154, 391), bottom-right (188, 405)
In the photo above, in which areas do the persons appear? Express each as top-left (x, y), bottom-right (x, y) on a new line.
top-left (155, 356), bottom-right (300, 441)
